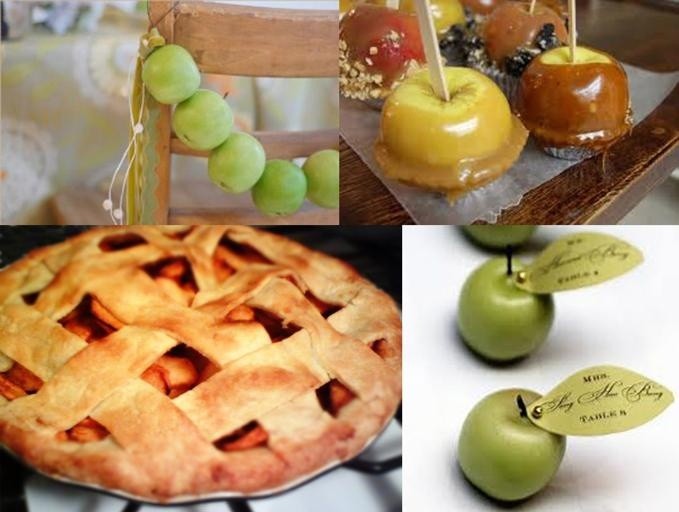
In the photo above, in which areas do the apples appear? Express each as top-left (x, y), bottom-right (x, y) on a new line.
top-left (457, 388), bottom-right (568, 503)
top-left (462, 225), bottom-right (539, 250)
top-left (141, 43), bottom-right (340, 220)
top-left (457, 256), bottom-right (554, 361)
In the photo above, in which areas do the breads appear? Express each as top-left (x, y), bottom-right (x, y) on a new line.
top-left (0, 225), bottom-right (403, 506)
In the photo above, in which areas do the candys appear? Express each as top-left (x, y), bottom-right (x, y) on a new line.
top-left (339, 1), bottom-right (633, 201)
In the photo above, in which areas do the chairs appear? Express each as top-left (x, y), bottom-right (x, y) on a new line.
top-left (134, 0), bottom-right (339, 225)
